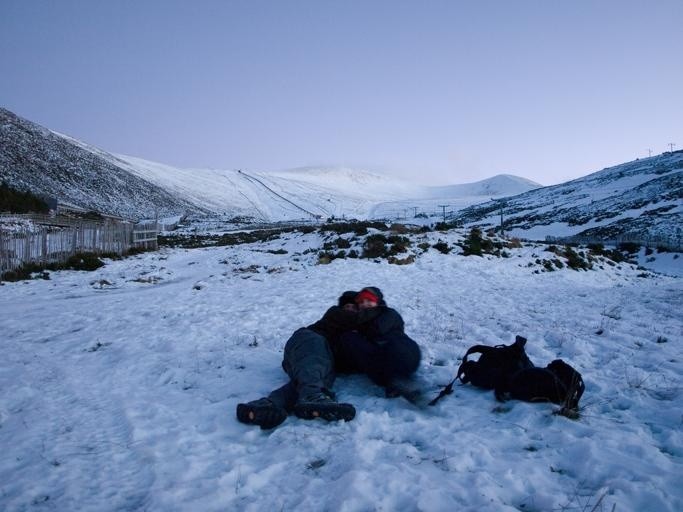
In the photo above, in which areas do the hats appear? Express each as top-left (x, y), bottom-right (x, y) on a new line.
top-left (339, 290), bottom-right (358, 303)
top-left (356, 286), bottom-right (383, 302)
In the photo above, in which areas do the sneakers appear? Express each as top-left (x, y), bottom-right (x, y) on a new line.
top-left (295, 394), bottom-right (357, 423)
top-left (236, 398), bottom-right (287, 429)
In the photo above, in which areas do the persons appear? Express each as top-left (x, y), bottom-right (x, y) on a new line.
top-left (235, 291), bottom-right (394, 430)
top-left (336, 286), bottom-right (423, 402)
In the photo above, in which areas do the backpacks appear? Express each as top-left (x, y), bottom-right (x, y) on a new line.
top-left (458, 337), bottom-right (535, 388)
top-left (495, 359), bottom-right (585, 416)
